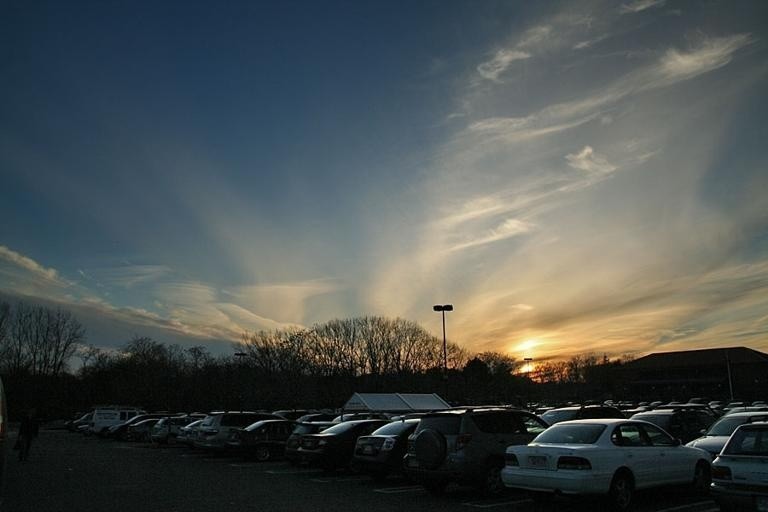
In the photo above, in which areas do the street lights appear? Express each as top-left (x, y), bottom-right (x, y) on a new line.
top-left (433, 303), bottom-right (454, 406)
top-left (234, 351), bottom-right (249, 413)
top-left (523, 358), bottom-right (533, 401)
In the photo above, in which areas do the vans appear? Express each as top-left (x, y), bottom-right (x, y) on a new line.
top-left (1, 375), bottom-right (8, 484)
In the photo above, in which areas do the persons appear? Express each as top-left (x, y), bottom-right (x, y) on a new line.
top-left (15, 406), bottom-right (40, 464)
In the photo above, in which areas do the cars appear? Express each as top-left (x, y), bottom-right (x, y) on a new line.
top-left (301, 418), bottom-right (393, 475)
top-left (351, 417), bottom-right (421, 471)
top-left (283, 419), bottom-right (338, 459)
top-left (711, 421), bottom-right (768, 512)
top-left (685, 411), bottom-right (768, 457)
top-left (403, 406), bottom-right (553, 500)
top-left (526, 400), bottom-right (768, 444)
top-left (499, 417), bottom-right (714, 508)
top-left (291, 408), bottom-right (426, 422)
top-left (61, 401), bottom-right (291, 468)
